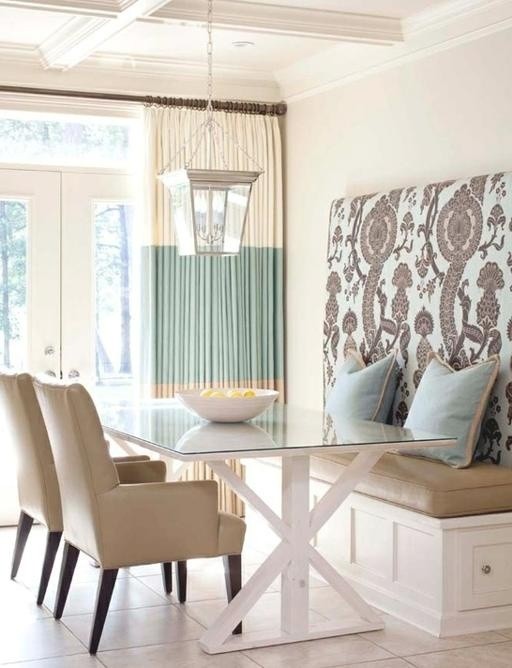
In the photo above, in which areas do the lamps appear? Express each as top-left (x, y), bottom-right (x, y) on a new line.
top-left (152, 1), bottom-right (267, 256)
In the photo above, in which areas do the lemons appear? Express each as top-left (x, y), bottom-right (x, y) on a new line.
top-left (200, 388), bottom-right (256, 398)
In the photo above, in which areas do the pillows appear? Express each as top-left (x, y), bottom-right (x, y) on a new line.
top-left (323, 345), bottom-right (401, 447)
top-left (398, 354), bottom-right (500, 472)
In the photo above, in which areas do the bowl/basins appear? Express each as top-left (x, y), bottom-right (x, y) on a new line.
top-left (175, 388), bottom-right (280, 424)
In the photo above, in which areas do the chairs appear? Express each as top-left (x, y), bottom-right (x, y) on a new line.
top-left (309, 169), bottom-right (512, 640)
top-left (34, 375), bottom-right (246, 658)
top-left (0, 368), bottom-right (172, 598)
